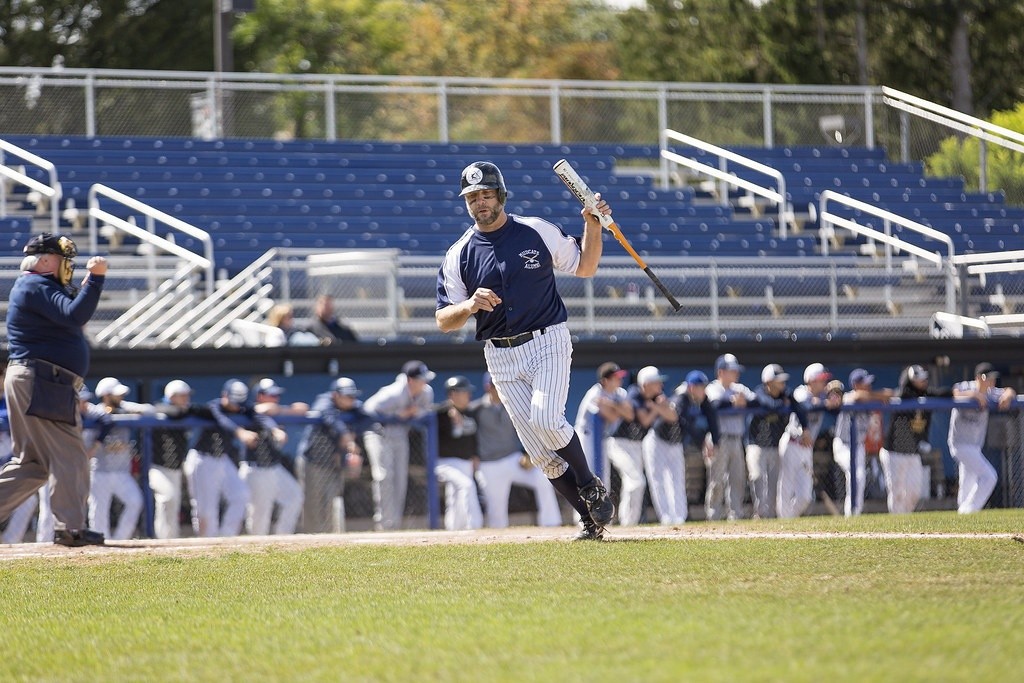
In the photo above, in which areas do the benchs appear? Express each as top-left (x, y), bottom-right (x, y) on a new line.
top-left (0, 134), bottom-right (1024, 338)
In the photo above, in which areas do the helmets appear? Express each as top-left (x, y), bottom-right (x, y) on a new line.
top-left (460, 161), bottom-right (508, 209)
top-left (22, 229), bottom-right (78, 258)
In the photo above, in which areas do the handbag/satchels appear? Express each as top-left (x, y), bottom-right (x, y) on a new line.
top-left (24, 359), bottom-right (76, 427)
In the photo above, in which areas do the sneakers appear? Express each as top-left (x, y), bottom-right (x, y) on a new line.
top-left (574, 513), bottom-right (612, 542)
top-left (578, 471), bottom-right (614, 526)
top-left (53, 529), bottom-right (104, 546)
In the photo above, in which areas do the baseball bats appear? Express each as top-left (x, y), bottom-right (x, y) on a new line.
top-left (553, 159), bottom-right (683, 311)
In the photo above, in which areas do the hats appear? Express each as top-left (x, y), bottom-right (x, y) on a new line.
top-left (715, 353), bottom-right (746, 372)
top-left (482, 372), bottom-right (494, 390)
top-left (222, 379), bottom-right (250, 403)
top-left (848, 368), bottom-right (873, 390)
top-left (329, 377), bottom-right (362, 397)
top-left (761, 363), bottom-right (790, 385)
top-left (596, 361), bottom-right (627, 383)
top-left (164, 379), bottom-right (192, 400)
top-left (402, 360), bottom-right (436, 381)
top-left (907, 363), bottom-right (928, 382)
top-left (95, 377), bottom-right (129, 398)
top-left (637, 366), bottom-right (669, 386)
top-left (974, 362), bottom-right (999, 378)
top-left (803, 362), bottom-right (831, 383)
top-left (444, 374), bottom-right (476, 391)
top-left (76, 383), bottom-right (93, 401)
top-left (685, 369), bottom-right (712, 385)
top-left (252, 377), bottom-right (286, 398)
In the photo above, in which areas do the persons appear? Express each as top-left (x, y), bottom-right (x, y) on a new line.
top-left (0, 233), bottom-right (108, 549)
top-left (0, 290), bottom-right (1016, 544)
top-left (435, 161), bottom-right (615, 542)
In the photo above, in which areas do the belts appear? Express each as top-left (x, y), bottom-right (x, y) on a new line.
top-left (492, 328), bottom-right (545, 348)
top-left (9, 358), bottom-right (85, 393)
